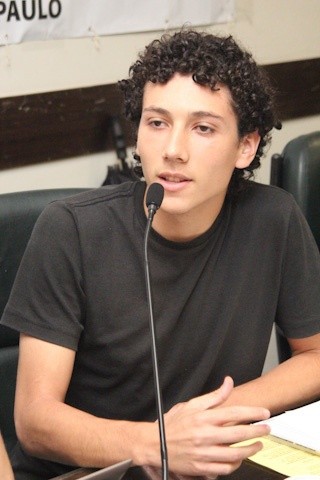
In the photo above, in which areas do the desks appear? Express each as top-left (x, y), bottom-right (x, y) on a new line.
top-left (52, 395), bottom-right (320, 480)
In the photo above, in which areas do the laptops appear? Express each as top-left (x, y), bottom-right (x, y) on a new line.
top-left (77, 458), bottom-right (133, 480)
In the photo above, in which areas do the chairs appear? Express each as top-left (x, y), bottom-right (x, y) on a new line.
top-left (0, 187), bottom-right (146, 480)
top-left (269, 130), bottom-right (320, 364)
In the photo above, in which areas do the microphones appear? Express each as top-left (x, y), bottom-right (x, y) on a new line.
top-left (142, 183), bottom-right (171, 479)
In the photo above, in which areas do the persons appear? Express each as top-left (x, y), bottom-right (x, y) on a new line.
top-left (2, 32), bottom-right (318, 475)
